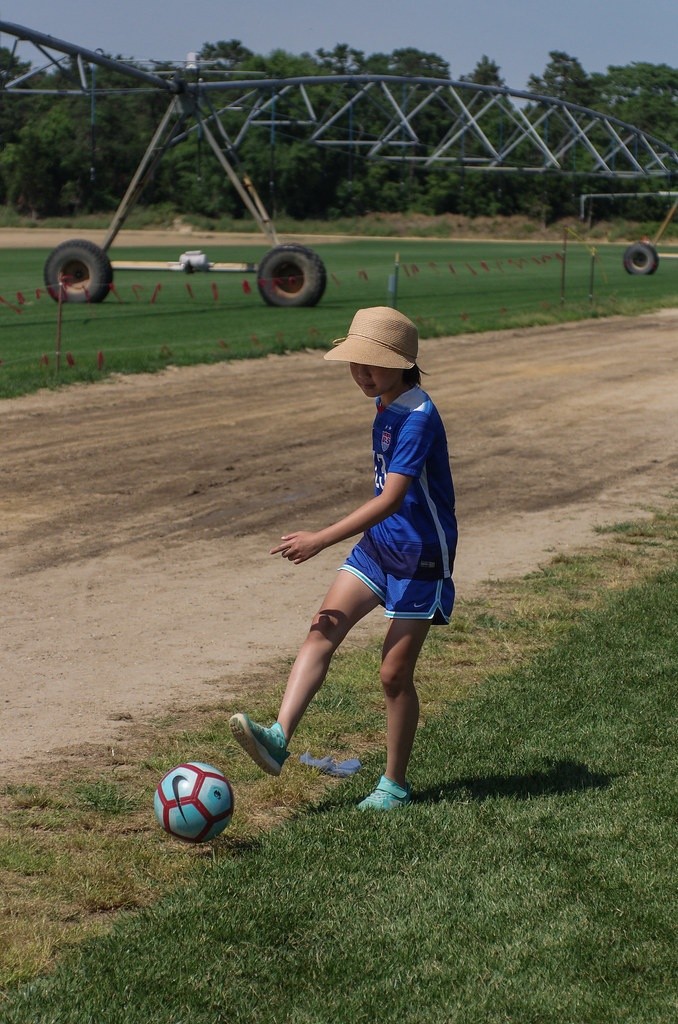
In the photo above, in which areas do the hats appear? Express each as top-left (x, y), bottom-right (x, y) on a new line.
top-left (323, 306), bottom-right (418, 369)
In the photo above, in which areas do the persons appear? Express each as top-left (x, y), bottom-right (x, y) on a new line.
top-left (228, 307), bottom-right (459, 813)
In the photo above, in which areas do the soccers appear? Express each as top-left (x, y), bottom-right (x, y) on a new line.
top-left (153, 760), bottom-right (236, 844)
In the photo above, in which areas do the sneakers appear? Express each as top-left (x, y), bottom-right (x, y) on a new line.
top-left (229, 713), bottom-right (291, 776)
top-left (356, 775), bottom-right (411, 815)
